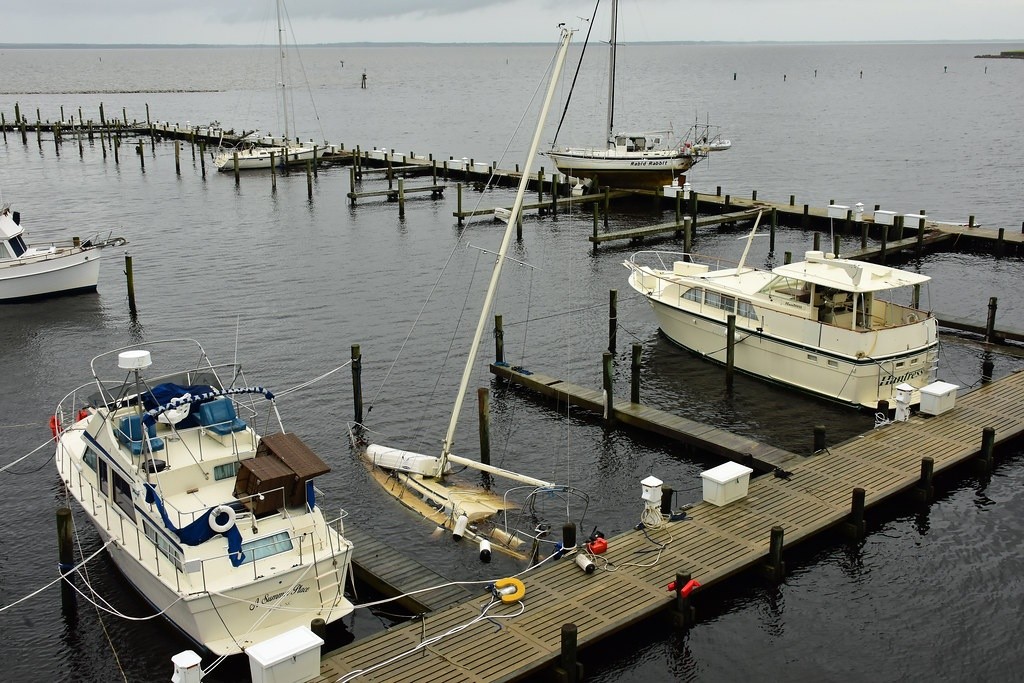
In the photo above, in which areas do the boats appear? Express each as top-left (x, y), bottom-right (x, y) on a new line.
top-left (52, 314), bottom-right (355, 658)
top-left (0, 204), bottom-right (129, 304)
top-left (618, 210), bottom-right (942, 414)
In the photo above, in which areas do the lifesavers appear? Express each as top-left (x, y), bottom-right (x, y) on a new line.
top-left (668, 578), bottom-right (702, 599)
top-left (208, 504), bottom-right (236, 533)
top-left (494, 577), bottom-right (526, 604)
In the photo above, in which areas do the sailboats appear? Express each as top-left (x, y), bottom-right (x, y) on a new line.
top-left (536, 1), bottom-right (732, 191)
top-left (211, 0), bottom-right (333, 172)
top-left (363, 24), bottom-right (580, 562)
